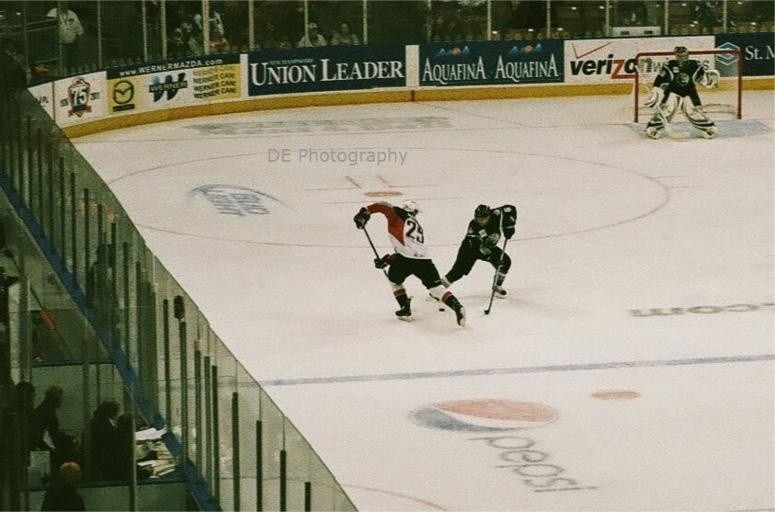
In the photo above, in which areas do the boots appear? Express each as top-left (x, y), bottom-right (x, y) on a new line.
top-left (450, 298), bottom-right (463, 325)
top-left (492, 283), bottom-right (506, 295)
top-left (396, 299), bottom-right (411, 316)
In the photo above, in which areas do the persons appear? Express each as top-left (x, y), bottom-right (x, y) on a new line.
top-left (44, 0), bottom-right (86, 75)
top-left (15, 242), bottom-right (154, 511)
top-left (429, 204), bottom-right (518, 301)
top-left (353, 198), bottom-right (465, 326)
top-left (643, 46), bottom-right (721, 141)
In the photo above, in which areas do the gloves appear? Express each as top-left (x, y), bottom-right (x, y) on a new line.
top-left (354, 207), bottom-right (370, 229)
top-left (374, 255), bottom-right (391, 268)
top-left (504, 227), bottom-right (514, 239)
top-left (493, 259), bottom-right (506, 269)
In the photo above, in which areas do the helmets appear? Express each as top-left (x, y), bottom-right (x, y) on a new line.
top-left (475, 204), bottom-right (491, 226)
top-left (403, 200), bottom-right (419, 216)
top-left (676, 46), bottom-right (688, 62)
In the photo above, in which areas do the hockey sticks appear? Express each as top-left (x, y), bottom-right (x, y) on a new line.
top-left (483, 237), bottom-right (507, 315)
top-left (634, 65), bottom-right (690, 139)
